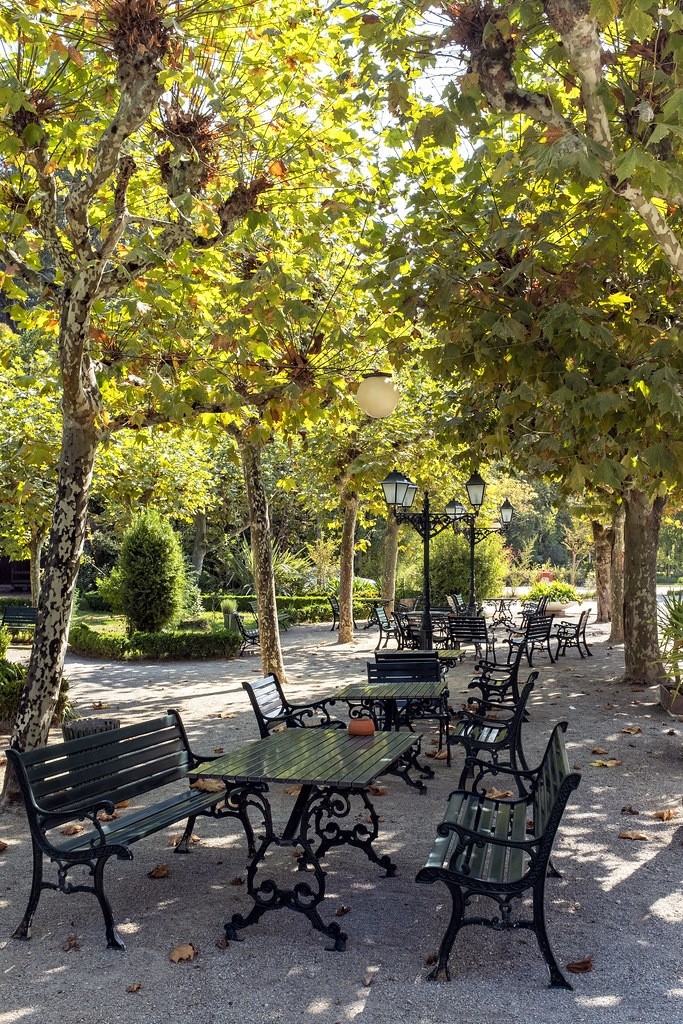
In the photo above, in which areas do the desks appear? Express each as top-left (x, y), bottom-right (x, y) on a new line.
top-left (401, 647), bottom-right (465, 680)
top-left (360, 599), bottom-right (393, 629)
top-left (184, 726), bottom-right (425, 954)
top-left (400, 611), bottom-right (450, 634)
top-left (331, 680), bottom-right (448, 778)
top-left (483, 598), bottom-right (520, 631)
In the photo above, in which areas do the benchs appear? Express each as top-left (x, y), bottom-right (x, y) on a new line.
top-left (5, 707), bottom-right (266, 949)
top-left (365, 652), bottom-right (458, 749)
top-left (413, 720), bottom-right (582, 992)
top-left (241, 671), bottom-right (347, 739)
top-left (374, 594), bottom-right (593, 668)
top-left (446, 651), bottom-right (540, 795)
top-left (248, 600), bottom-right (291, 632)
top-left (328, 597), bottom-right (357, 630)
top-left (232, 610), bottom-right (260, 657)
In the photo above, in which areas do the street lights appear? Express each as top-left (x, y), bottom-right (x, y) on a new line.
top-left (443, 496), bottom-right (515, 636)
top-left (381, 466), bottom-right (487, 649)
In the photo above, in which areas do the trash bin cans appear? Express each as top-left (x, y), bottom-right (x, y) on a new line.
top-left (538, 570), bottom-right (552, 584)
top-left (61, 716), bottom-right (121, 799)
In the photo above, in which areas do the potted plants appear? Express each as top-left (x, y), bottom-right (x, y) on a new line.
top-left (648, 584), bottom-right (683, 714)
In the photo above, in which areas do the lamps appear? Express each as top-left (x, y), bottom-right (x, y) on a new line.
top-left (378, 466), bottom-right (512, 645)
top-left (356, 367), bottom-right (402, 417)
